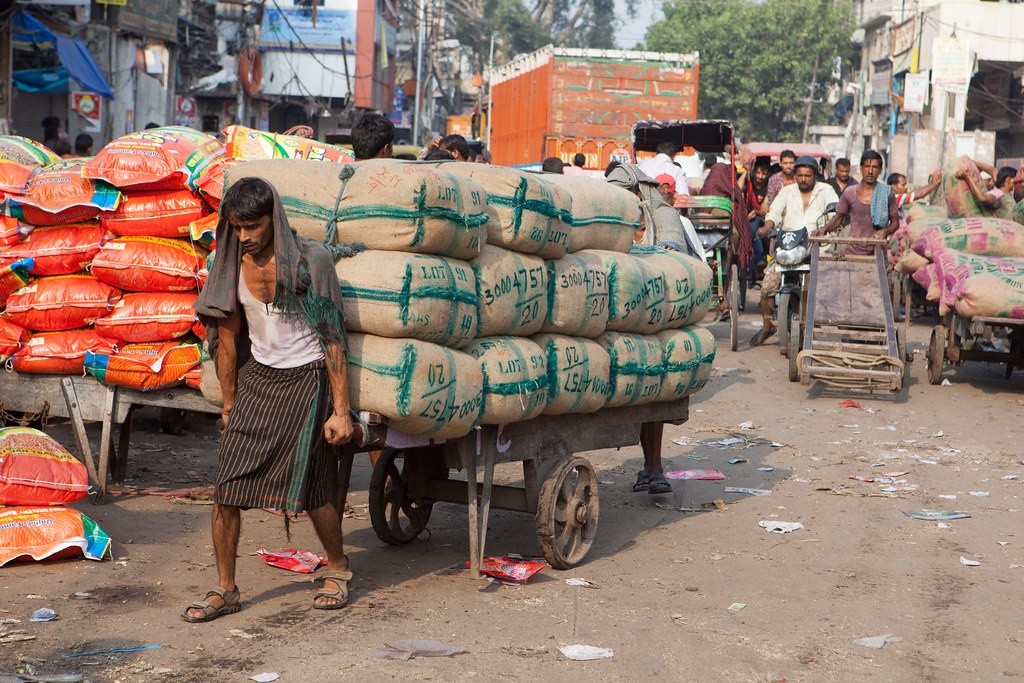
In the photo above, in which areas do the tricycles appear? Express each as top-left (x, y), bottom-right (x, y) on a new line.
top-left (631, 120), bottom-right (745, 352)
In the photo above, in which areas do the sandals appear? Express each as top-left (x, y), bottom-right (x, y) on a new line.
top-left (181, 584), bottom-right (241, 622)
top-left (313, 555), bottom-right (353, 608)
top-left (633, 471), bottom-right (650, 492)
top-left (649, 471), bottom-right (673, 493)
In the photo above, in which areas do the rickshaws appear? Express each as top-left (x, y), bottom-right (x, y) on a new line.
top-left (781, 228), bottom-right (915, 393)
top-left (215, 396), bottom-right (696, 571)
top-left (925, 308), bottom-right (1024, 384)
top-left (0, 364), bottom-right (225, 504)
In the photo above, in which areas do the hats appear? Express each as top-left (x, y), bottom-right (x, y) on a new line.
top-left (655, 173), bottom-right (675, 193)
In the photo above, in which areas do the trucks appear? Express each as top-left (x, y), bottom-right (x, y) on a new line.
top-left (489, 44), bottom-right (700, 180)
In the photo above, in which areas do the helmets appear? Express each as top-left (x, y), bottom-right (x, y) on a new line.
top-left (794, 155), bottom-right (819, 172)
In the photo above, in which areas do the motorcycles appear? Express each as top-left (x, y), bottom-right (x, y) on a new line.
top-left (754, 201), bottom-right (839, 350)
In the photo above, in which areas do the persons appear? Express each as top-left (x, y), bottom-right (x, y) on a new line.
top-left (810, 150), bottom-right (900, 346)
top-left (604, 142), bottom-right (1017, 321)
top-left (41, 116), bottom-right (71, 157)
top-left (563, 154), bottom-right (585, 175)
top-left (182, 177), bottom-right (355, 623)
top-left (605, 163), bottom-right (703, 493)
top-left (351, 114), bottom-right (395, 161)
top-left (655, 173), bottom-right (707, 262)
top-left (416, 135), bottom-right (484, 162)
top-left (543, 157), bottom-right (564, 174)
top-left (749, 155), bottom-right (839, 346)
top-left (75, 134), bottom-right (94, 157)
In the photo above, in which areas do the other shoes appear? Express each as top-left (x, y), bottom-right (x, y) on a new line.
top-left (757, 262), bottom-right (765, 272)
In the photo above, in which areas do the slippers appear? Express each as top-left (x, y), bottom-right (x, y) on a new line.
top-left (750, 327), bottom-right (777, 345)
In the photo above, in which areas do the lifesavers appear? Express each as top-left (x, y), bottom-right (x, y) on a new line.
top-left (238, 46), bottom-right (263, 97)
